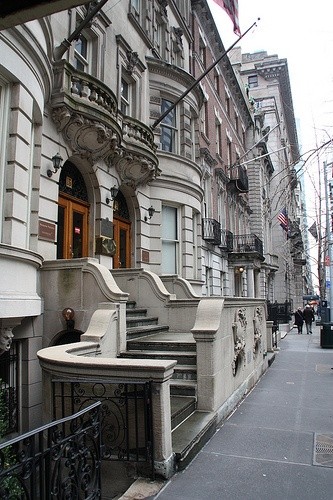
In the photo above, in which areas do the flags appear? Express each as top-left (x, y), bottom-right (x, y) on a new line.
top-left (278, 207), bottom-right (317, 241)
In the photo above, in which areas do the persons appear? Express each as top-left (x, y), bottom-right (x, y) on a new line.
top-left (295, 304), bottom-right (315, 335)
